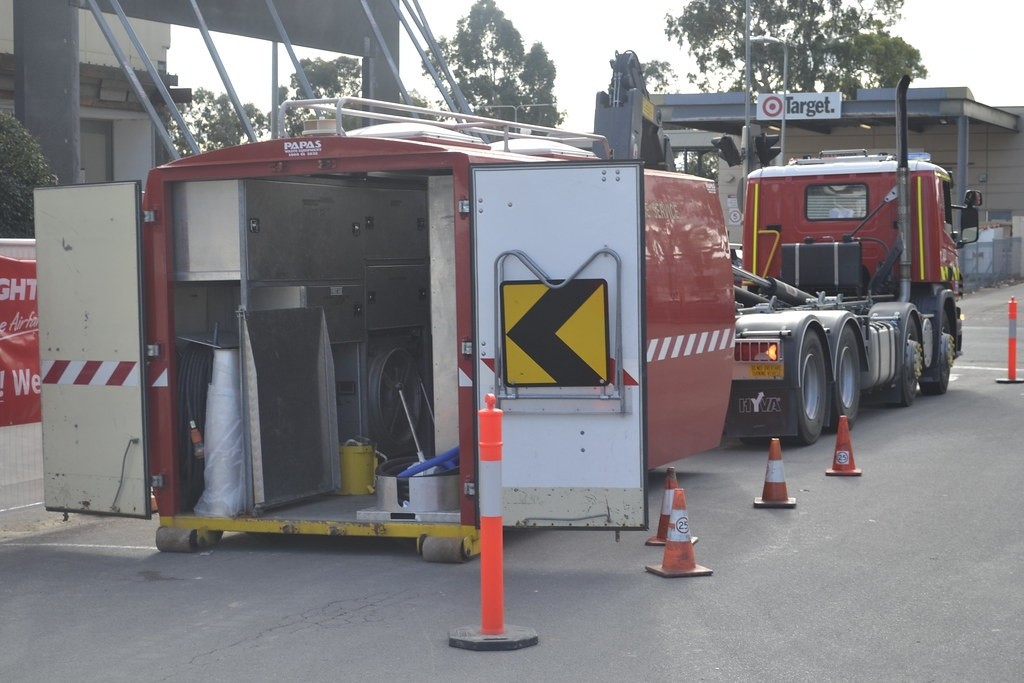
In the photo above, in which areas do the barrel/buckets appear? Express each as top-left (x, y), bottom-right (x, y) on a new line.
top-left (341, 442), bottom-right (376, 496)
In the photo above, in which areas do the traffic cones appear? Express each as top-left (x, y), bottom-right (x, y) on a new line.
top-left (754, 438), bottom-right (796, 508)
top-left (645, 488), bottom-right (713, 578)
top-left (825, 415), bottom-right (862, 476)
top-left (645, 467), bottom-right (698, 546)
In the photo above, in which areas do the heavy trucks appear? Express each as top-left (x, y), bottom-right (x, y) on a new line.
top-left (683, 76), bottom-right (982, 445)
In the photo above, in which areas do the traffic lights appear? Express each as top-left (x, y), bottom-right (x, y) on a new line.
top-left (755, 131), bottom-right (781, 166)
top-left (710, 137), bottom-right (739, 167)
